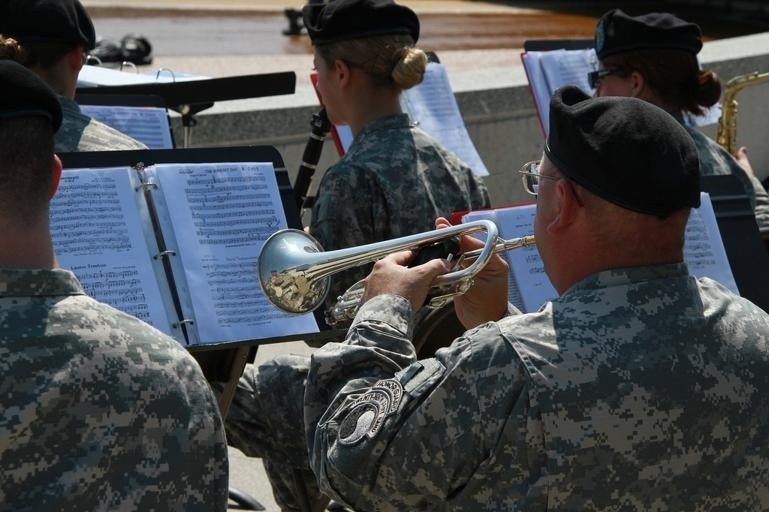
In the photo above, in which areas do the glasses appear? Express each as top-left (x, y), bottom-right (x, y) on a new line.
top-left (517, 159), bottom-right (584, 210)
top-left (586, 65), bottom-right (631, 90)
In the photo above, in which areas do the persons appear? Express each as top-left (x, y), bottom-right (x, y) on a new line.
top-left (224, 2), bottom-right (493, 512)
top-left (0, 56), bottom-right (230, 512)
top-left (588, 9), bottom-right (756, 217)
top-left (733, 146), bottom-right (769, 242)
top-left (303, 85), bottom-right (769, 512)
top-left (0, 0), bottom-right (150, 154)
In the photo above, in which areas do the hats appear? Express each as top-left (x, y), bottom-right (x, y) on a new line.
top-left (301, 0), bottom-right (420, 47)
top-left (0, 59), bottom-right (64, 170)
top-left (0, 0), bottom-right (97, 62)
top-left (592, 7), bottom-right (704, 61)
top-left (543, 84), bottom-right (702, 219)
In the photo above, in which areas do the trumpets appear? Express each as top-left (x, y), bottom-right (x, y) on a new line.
top-left (258, 219), bottom-right (537, 325)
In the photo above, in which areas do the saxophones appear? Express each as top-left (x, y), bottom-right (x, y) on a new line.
top-left (718, 71), bottom-right (768, 156)
